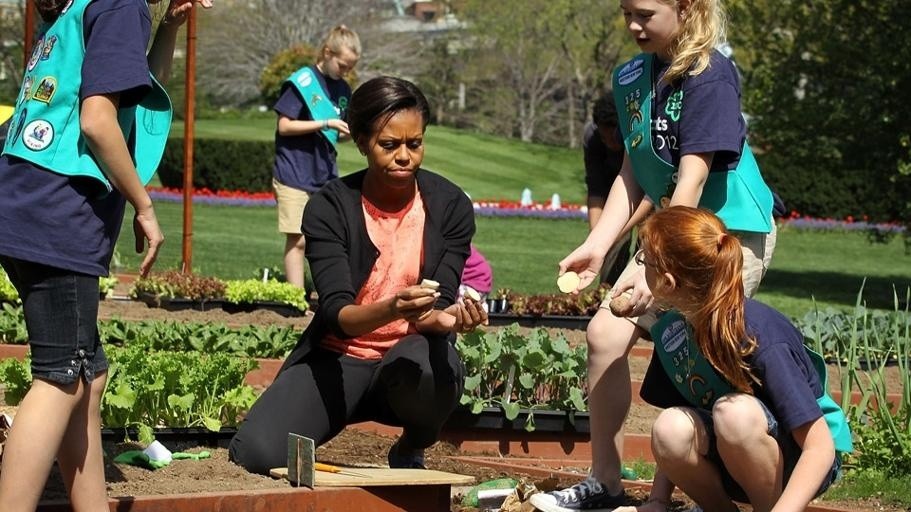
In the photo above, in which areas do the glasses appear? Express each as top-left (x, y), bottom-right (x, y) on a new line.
top-left (635, 250), bottom-right (657, 269)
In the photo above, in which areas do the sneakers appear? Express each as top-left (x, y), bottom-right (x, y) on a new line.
top-left (530, 476), bottom-right (625, 512)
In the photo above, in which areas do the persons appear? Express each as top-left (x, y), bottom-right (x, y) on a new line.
top-left (272, 24), bottom-right (362, 294)
top-left (581, 91), bottom-right (643, 290)
top-left (457, 246), bottom-right (494, 301)
top-left (611, 205), bottom-right (857, 512)
top-left (529, 0), bottom-right (778, 512)
top-left (0, 1), bottom-right (215, 511)
top-left (227, 75), bottom-right (488, 478)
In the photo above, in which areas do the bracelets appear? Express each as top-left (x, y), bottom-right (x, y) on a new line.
top-left (323, 120), bottom-right (331, 132)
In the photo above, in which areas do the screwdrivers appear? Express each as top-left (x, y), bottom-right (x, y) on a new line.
top-left (314, 462), bottom-right (372, 478)
